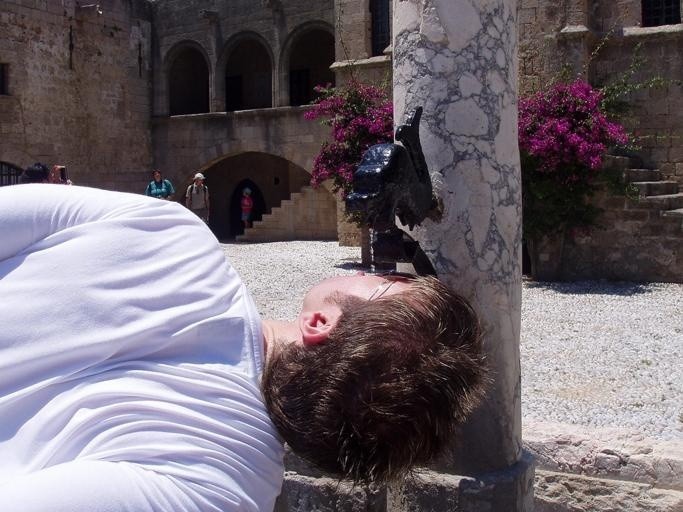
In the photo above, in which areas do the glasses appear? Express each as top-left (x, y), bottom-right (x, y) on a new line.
top-left (368, 269), bottom-right (414, 300)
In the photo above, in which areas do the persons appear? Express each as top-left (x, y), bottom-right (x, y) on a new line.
top-left (144, 169), bottom-right (174, 202)
top-left (184, 173), bottom-right (212, 226)
top-left (0, 180), bottom-right (495, 511)
top-left (46, 165), bottom-right (74, 186)
top-left (240, 187), bottom-right (253, 228)
top-left (14, 161), bottom-right (48, 185)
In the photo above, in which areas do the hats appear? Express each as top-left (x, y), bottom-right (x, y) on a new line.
top-left (195, 172), bottom-right (203, 179)
